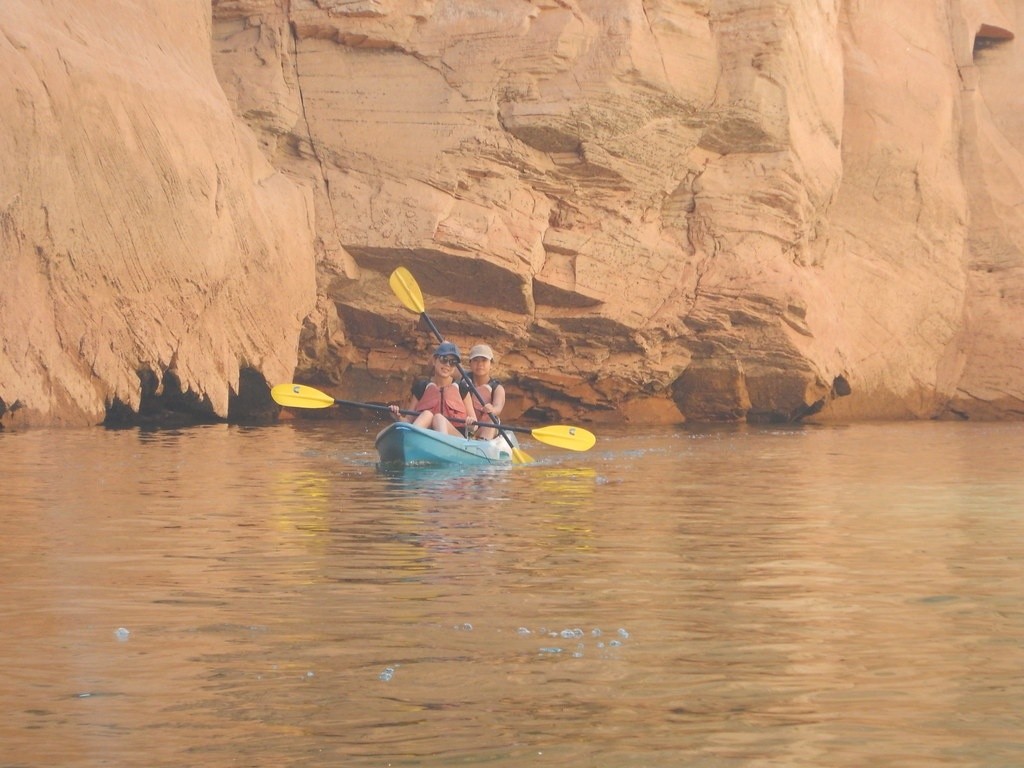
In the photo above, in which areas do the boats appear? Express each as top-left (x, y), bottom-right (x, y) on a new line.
top-left (372, 422), bottom-right (522, 474)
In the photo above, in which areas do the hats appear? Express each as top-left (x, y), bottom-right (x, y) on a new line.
top-left (433, 343), bottom-right (461, 363)
top-left (469, 345), bottom-right (493, 361)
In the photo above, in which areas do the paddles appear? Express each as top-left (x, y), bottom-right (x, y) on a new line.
top-left (388, 266), bottom-right (535, 465)
top-left (270, 382), bottom-right (596, 454)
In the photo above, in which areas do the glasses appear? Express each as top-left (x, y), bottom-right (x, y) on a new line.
top-left (439, 355), bottom-right (458, 367)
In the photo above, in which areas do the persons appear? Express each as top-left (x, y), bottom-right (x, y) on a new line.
top-left (388, 341), bottom-right (505, 441)
